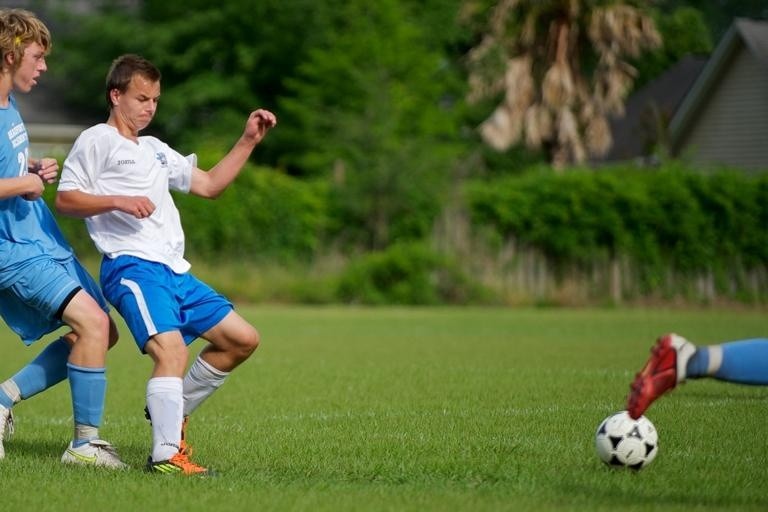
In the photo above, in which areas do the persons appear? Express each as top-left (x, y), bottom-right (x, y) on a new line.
top-left (0, 9), bottom-right (127, 472)
top-left (54, 53), bottom-right (278, 477)
top-left (626, 329), bottom-right (766, 421)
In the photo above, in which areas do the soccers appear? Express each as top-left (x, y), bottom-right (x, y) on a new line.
top-left (596, 411), bottom-right (659, 470)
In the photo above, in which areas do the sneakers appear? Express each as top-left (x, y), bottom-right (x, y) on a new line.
top-left (146, 454), bottom-right (210, 478)
top-left (0, 402), bottom-right (12, 456)
top-left (143, 402), bottom-right (189, 454)
top-left (626, 332), bottom-right (697, 420)
top-left (60, 438), bottom-right (130, 471)
